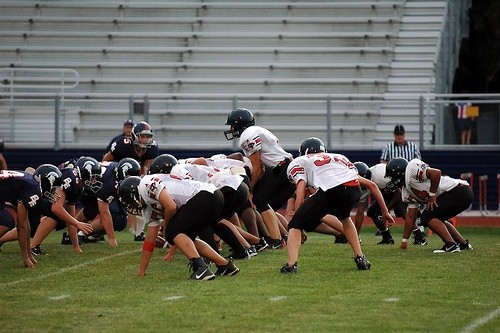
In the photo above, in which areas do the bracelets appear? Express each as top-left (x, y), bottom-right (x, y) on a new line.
top-left (429, 191), bottom-right (437, 197)
top-left (402, 239), bottom-right (409, 243)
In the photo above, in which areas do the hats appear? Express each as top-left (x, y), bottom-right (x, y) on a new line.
top-left (394, 124), bottom-right (405, 134)
top-left (123, 119), bottom-right (134, 126)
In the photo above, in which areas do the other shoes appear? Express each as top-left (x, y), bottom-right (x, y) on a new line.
top-left (335, 233), bottom-right (348, 244)
top-left (60, 233), bottom-right (82, 245)
top-left (412, 238), bottom-right (429, 246)
top-left (214, 259), bottom-right (240, 278)
top-left (376, 237), bottom-right (395, 245)
top-left (187, 265), bottom-right (216, 281)
top-left (77, 234), bottom-right (97, 243)
top-left (432, 241), bottom-right (461, 253)
top-left (456, 239), bottom-right (474, 251)
top-left (353, 254), bottom-right (372, 270)
top-left (278, 261), bottom-right (299, 274)
top-left (224, 236), bottom-right (284, 261)
top-left (134, 231), bottom-right (146, 241)
top-left (155, 235), bottom-right (170, 249)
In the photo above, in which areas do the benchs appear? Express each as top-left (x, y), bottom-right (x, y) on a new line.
top-left (0, 0), bottom-right (472, 149)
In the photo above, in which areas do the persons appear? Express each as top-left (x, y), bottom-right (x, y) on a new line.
top-left (0, 108), bottom-right (394, 282)
top-left (226, 109), bottom-right (298, 250)
top-left (383, 153), bottom-right (476, 252)
top-left (114, 168), bottom-right (240, 281)
top-left (380, 124), bottom-right (422, 167)
top-left (351, 159), bottom-right (429, 246)
top-left (454, 102), bottom-right (476, 144)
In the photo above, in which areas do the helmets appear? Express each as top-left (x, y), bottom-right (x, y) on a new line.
top-left (131, 121), bottom-right (153, 148)
top-left (354, 161), bottom-right (372, 179)
top-left (223, 107), bottom-right (254, 140)
top-left (34, 164), bottom-right (63, 197)
top-left (298, 136), bottom-right (326, 156)
top-left (385, 157), bottom-right (409, 188)
top-left (117, 158), bottom-right (142, 183)
top-left (74, 157), bottom-right (102, 194)
top-left (117, 176), bottom-right (147, 210)
top-left (147, 155), bottom-right (178, 175)
